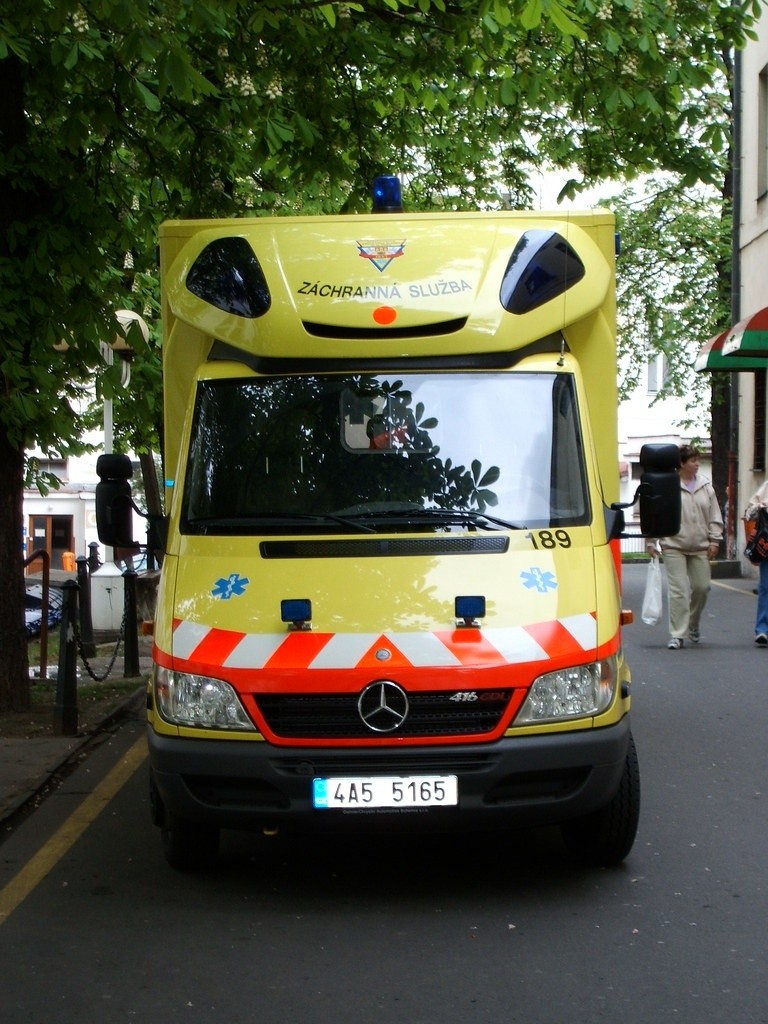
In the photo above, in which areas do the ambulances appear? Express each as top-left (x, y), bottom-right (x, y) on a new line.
top-left (140, 211), bottom-right (644, 869)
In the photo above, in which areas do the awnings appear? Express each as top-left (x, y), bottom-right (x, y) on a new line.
top-left (721, 307), bottom-right (768, 358)
top-left (694, 330), bottom-right (768, 372)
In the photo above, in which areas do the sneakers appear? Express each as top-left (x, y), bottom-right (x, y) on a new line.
top-left (689, 626), bottom-right (699, 642)
top-left (667, 638), bottom-right (681, 649)
top-left (755, 630), bottom-right (767, 645)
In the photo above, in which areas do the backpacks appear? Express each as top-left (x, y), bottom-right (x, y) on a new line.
top-left (741, 507), bottom-right (768, 566)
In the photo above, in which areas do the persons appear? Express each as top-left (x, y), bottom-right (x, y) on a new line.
top-left (744, 481), bottom-right (768, 649)
top-left (646, 445), bottom-right (724, 649)
